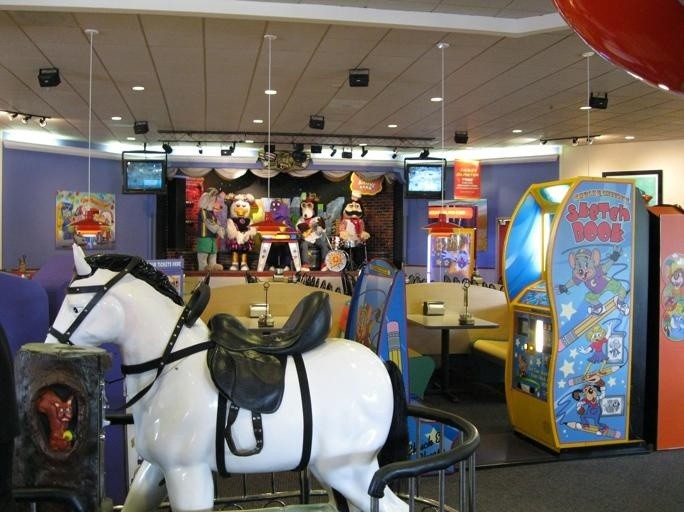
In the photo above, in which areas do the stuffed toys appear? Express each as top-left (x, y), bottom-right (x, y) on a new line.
top-left (337, 197), bottom-right (371, 268)
top-left (294, 191), bottom-right (329, 272)
top-left (226, 192), bottom-right (257, 270)
top-left (193, 186), bottom-right (224, 271)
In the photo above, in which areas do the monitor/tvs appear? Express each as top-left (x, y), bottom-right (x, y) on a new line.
top-left (121, 159), bottom-right (168, 194)
top-left (403, 158), bottom-right (446, 200)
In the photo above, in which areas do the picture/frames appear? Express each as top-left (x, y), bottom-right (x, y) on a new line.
top-left (602, 170), bottom-right (663, 208)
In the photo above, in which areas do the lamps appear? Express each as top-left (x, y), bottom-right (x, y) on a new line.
top-left (8, 113), bottom-right (47, 128)
top-left (66, 29), bottom-right (110, 239)
top-left (219, 141), bottom-right (235, 156)
top-left (329, 145), bottom-right (399, 160)
top-left (541, 136), bottom-right (592, 147)
top-left (418, 41), bottom-right (463, 239)
top-left (246, 34), bottom-right (288, 235)
top-left (162, 141), bottom-right (172, 154)
